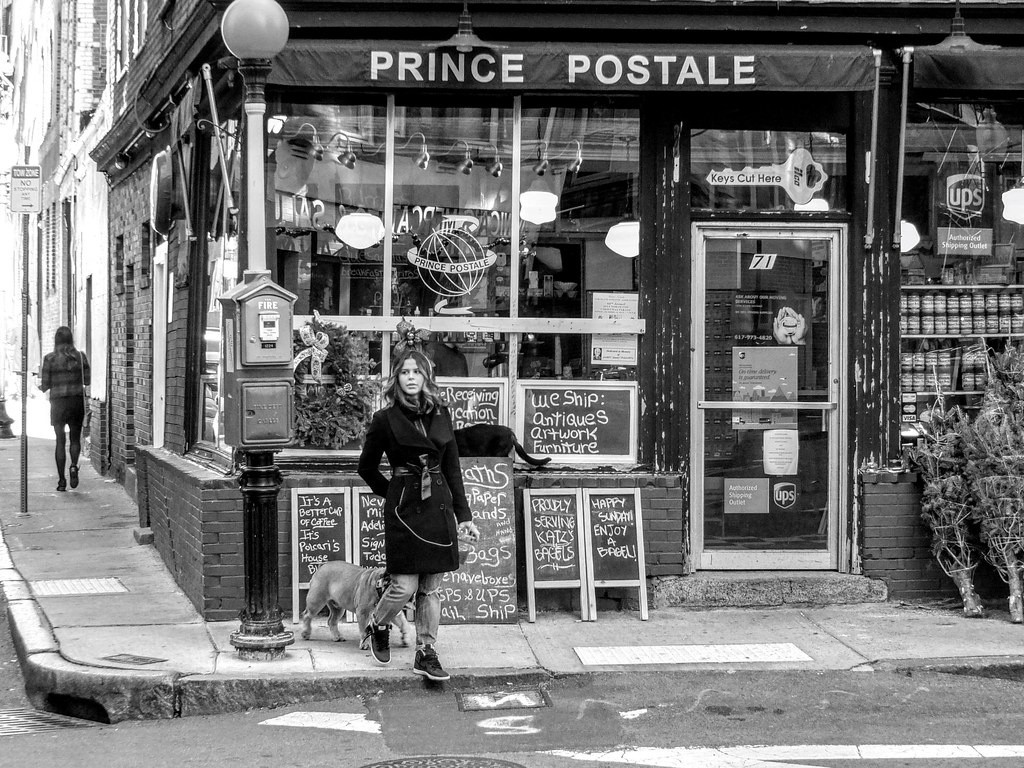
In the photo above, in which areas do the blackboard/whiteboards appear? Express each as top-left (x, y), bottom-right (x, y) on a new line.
top-left (435, 457), bottom-right (521, 625)
top-left (432, 375), bottom-right (509, 429)
top-left (584, 486), bottom-right (649, 589)
top-left (523, 487), bottom-right (588, 591)
top-left (514, 378), bottom-right (641, 465)
top-left (291, 486), bottom-right (353, 589)
top-left (351, 486), bottom-right (388, 570)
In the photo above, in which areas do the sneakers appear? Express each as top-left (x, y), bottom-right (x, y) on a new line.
top-left (413, 644), bottom-right (450, 680)
top-left (362, 613), bottom-right (393, 665)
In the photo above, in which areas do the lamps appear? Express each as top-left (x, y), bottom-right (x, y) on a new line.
top-left (605, 136), bottom-right (639, 258)
top-left (904, 1), bottom-right (1004, 52)
top-left (335, 143), bottom-right (386, 250)
top-left (280, 122), bottom-right (582, 176)
top-left (422, 3), bottom-right (509, 53)
top-left (794, 133), bottom-right (830, 211)
top-left (520, 118), bottom-right (559, 226)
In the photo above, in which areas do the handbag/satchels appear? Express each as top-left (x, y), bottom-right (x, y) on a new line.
top-left (83, 409), bottom-right (92, 427)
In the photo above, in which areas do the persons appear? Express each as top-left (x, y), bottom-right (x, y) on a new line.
top-left (37, 326), bottom-right (92, 492)
top-left (356, 351), bottom-right (481, 681)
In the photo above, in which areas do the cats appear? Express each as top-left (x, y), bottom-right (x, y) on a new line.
top-left (453, 424), bottom-right (551, 466)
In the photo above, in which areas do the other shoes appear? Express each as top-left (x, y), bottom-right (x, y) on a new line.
top-left (57, 480), bottom-right (66, 491)
top-left (69, 465), bottom-right (78, 488)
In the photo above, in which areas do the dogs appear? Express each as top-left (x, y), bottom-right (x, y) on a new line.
top-left (301, 561), bottom-right (415, 647)
